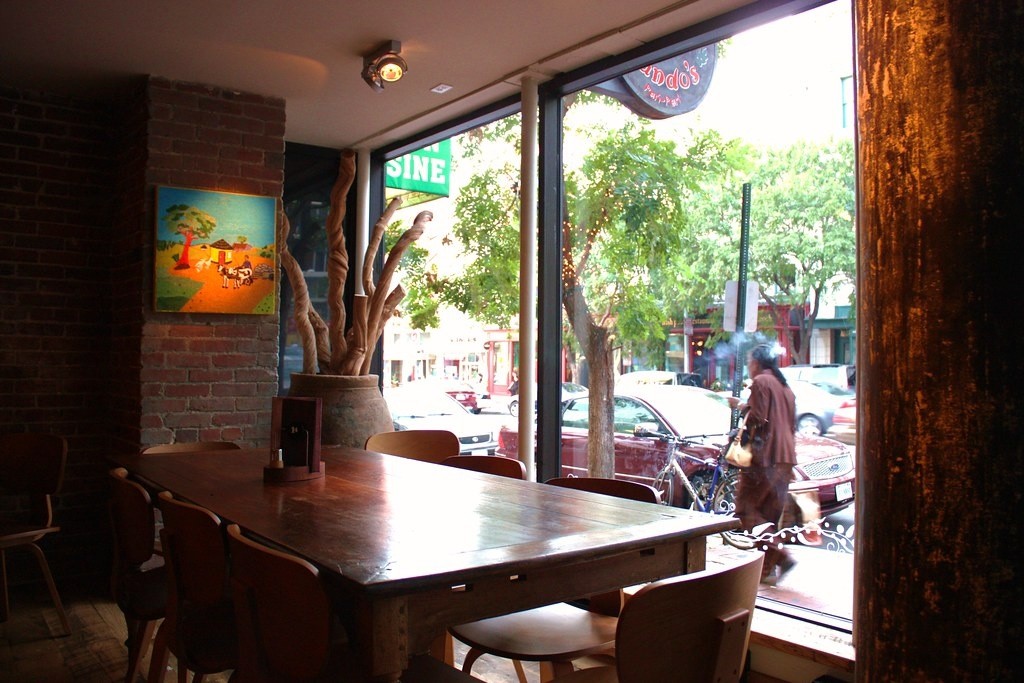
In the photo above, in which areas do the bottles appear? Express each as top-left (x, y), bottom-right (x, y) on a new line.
top-left (271, 448), bottom-right (284, 468)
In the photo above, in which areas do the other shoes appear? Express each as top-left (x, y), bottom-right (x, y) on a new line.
top-left (764, 555), bottom-right (798, 585)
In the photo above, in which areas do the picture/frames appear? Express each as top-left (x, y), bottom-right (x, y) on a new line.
top-left (154, 182), bottom-right (277, 316)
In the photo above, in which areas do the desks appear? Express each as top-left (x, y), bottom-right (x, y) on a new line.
top-left (108, 445), bottom-right (744, 683)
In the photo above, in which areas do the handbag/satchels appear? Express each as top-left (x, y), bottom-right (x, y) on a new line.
top-left (725, 410), bottom-right (755, 469)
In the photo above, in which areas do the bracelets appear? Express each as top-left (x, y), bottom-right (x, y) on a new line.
top-left (736, 402), bottom-right (744, 410)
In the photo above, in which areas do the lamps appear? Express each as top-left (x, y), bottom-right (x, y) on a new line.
top-left (361, 39), bottom-right (409, 92)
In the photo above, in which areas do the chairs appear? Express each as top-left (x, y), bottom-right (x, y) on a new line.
top-left (147, 491), bottom-right (235, 683)
top-left (445, 477), bottom-right (662, 678)
top-left (0, 433), bottom-right (72, 638)
top-left (364, 430), bottom-right (460, 464)
top-left (227, 524), bottom-right (487, 683)
top-left (545, 550), bottom-right (765, 683)
top-left (139, 441), bottom-right (244, 454)
top-left (438, 454), bottom-right (528, 481)
top-left (105, 467), bottom-right (172, 683)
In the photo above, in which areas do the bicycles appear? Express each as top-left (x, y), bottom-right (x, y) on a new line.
top-left (635, 429), bottom-right (759, 551)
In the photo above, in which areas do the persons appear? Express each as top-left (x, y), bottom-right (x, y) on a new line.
top-left (727, 343), bottom-right (799, 586)
top-left (510, 371), bottom-right (518, 396)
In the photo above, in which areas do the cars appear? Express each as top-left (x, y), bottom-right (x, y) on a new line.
top-left (621, 370), bottom-right (705, 389)
top-left (398, 377), bottom-right (492, 415)
top-left (381, 387), bottom-right (499, 456)
top-left (507, 382), bottom-right (589, 417)
top-left (496, 385), bottom-right (855, 545)
top-left (779, 364), bottom-right (856, 391)
top-left (717, 380), bottom-right (856, 447)
